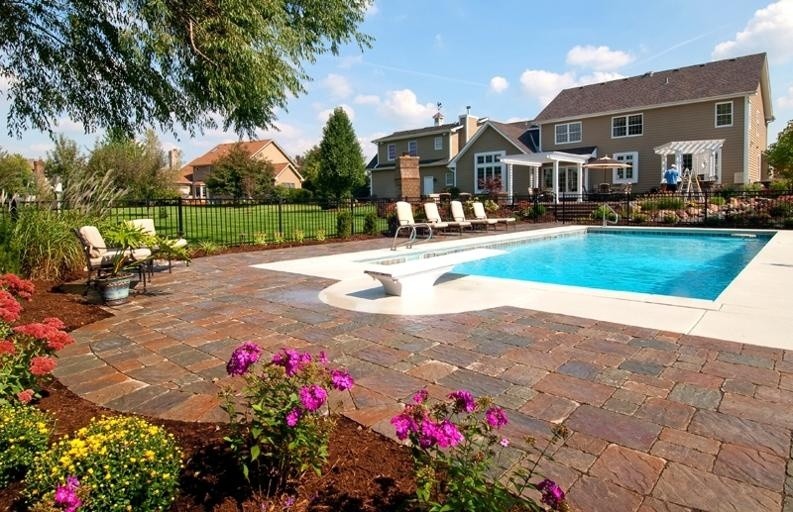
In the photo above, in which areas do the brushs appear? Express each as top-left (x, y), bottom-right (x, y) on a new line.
top-left (678, 168), bottom-right (705, 202)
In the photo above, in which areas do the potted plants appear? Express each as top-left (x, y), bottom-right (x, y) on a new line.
top-left (90, 221), bottom-right (192, 307)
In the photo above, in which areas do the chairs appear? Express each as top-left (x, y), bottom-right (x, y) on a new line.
top-left (395, 199), bottom-right (517, 240)
top-left (69, 216), bottom-right (190, 294)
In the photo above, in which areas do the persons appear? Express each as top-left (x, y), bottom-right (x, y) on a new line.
top-left (663, 164), bottom-right (680, 191)
